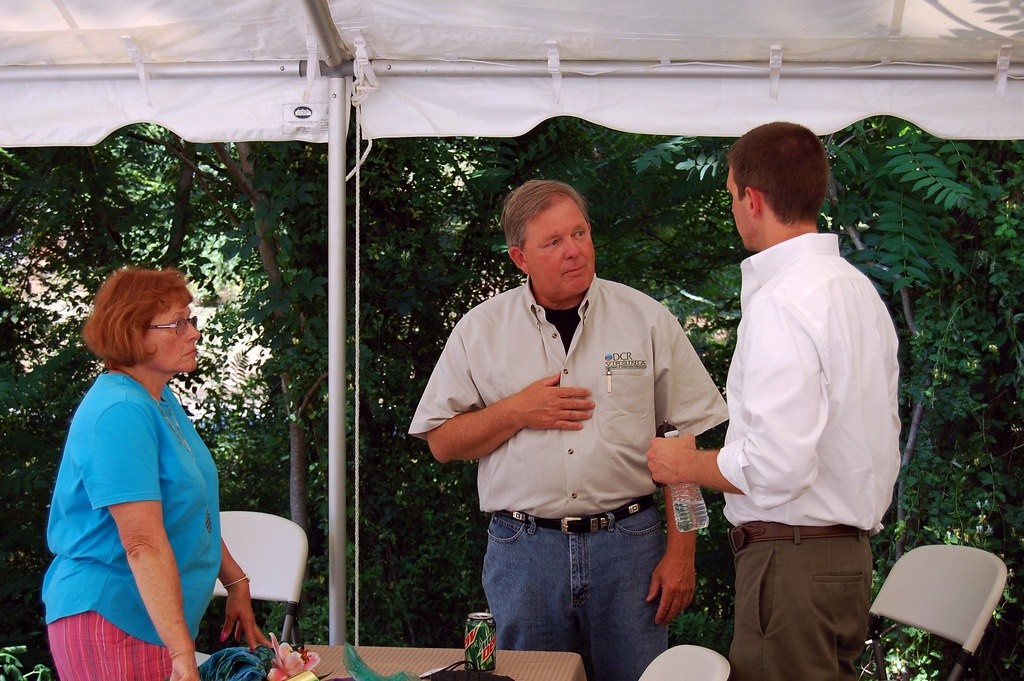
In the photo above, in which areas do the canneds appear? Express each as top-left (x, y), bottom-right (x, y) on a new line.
top-left (464, 612), bottom-right (496, 673)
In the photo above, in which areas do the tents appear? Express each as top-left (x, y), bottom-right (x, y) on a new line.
top-left (1, 2), bottom-right (1023, 641)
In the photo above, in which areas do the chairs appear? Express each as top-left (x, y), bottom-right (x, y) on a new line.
top-left (191, 510), bottom-right (309, 665)
top-left (640, 644), bottom-right (732, 681)
top-left (868, 544), bottom-right (1007, 681)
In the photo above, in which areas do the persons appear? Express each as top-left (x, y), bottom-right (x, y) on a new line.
top-left (409, 180), bottom-right (729, 681)
top-left (40, 266), bottom-right (274, 681)
top-left (646, 121), bottom-right (903, 680)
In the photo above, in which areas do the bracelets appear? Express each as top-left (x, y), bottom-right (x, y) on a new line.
top-left (223, 572), bottom-right (250, 588)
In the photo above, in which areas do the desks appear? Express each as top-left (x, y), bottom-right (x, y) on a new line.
top-left (290, 644), bottom-right (587, 681)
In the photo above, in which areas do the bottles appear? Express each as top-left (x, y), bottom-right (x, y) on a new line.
top-left (665, 430), bottom-right (709, 533)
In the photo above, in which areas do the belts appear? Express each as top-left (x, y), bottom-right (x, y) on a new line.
top-left (495, 494), bottom-right (654, 535)
top-left (730, 521), bottom-right (868, 550)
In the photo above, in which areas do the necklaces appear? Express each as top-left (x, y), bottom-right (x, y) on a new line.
top-left (149, 391), bottom-right (195, 463)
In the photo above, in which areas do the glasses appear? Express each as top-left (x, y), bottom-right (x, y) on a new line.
top-left (148, 316), bottom-right (198, 335)
